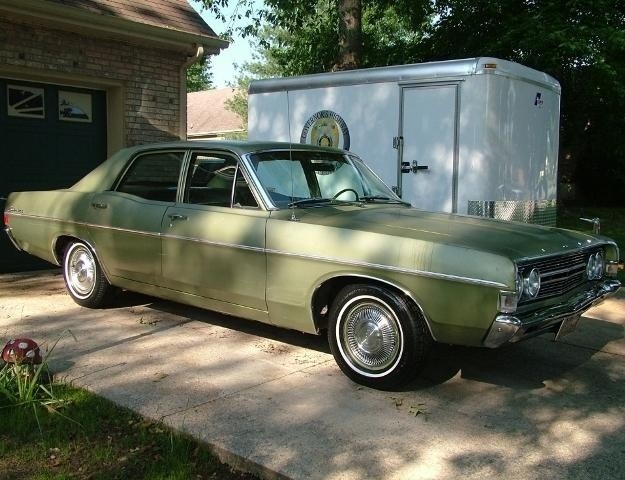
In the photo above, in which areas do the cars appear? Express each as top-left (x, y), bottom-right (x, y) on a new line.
top-left (0, 133), bottom-right (621, 391)
